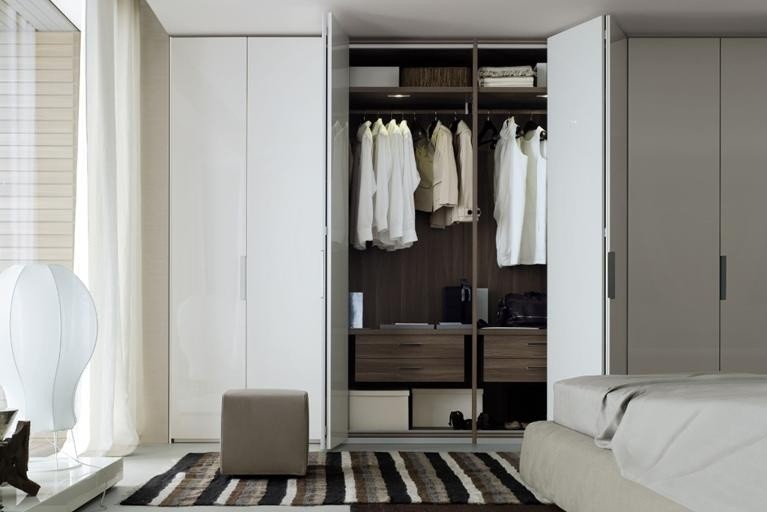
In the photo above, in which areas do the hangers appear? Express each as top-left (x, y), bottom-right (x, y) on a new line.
top-left (361, 110), bottom-right (538, 136)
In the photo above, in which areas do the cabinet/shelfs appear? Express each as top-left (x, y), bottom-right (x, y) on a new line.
top-left (628, 38), bottom-right (767, 374)
top-left (169, 36), bottom-right (325, 443)
top-left (325, 13), bottom-right (628, 450)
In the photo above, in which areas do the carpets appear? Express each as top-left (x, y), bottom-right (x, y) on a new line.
top-left (350, 503), bottom-right (566, 512)
top-left (114, 452), bottom-right (555, 506)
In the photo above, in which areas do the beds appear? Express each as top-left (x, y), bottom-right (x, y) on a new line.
top-left (519, 373), bottom-right (766, 512)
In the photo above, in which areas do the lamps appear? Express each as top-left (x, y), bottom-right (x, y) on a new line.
top-left (0, 263), bottom-right (98, 474)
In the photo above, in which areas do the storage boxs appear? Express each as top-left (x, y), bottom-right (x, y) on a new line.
top-left (411, 388), bottom-right (484, 427)
top-left (349, 390), bottom-right (410, 434)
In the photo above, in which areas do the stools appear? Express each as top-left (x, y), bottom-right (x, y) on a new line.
top-left (221, 389), bottom-right (309, 477)
